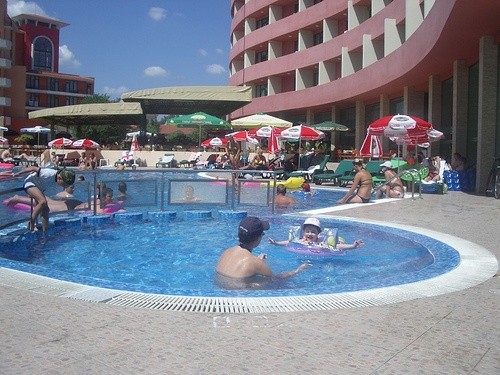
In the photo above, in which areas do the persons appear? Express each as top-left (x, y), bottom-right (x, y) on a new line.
top-left (337, 160), bottom-right (372, 203)
top-left (375, 161), bottom-right (404, 199)
top-left (269, 185), bottom-right (295, 206)
top-left (215, 216), bottom-right (311, 288)
top-left (267, 217), bottom-right (363, 256)
top-left (13, 168), bottom-right (75, 242)
top-left (0, 133), bottom-right (470, 212)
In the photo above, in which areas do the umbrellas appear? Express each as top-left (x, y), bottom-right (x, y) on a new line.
top-left (231, 114), bottom-right (294, 129)
top-left (225, 131), bottom-right (260, 143)
top-left (72, 138), bottom-right (99, 147)
top-left (368, 115), bottom-right (443, 143)
top-left (48, 138), bottom-right (73, 146)
top-left (166, 112), bottom-right (228, 124)
top-left (247, 127), bottom-right (285, 137)
top-left (282, 126), bottom-right (320, 139)
top-left (200, 137), bottom-right (227, 147)
top-left (310, 120), bottom-right (351, 132)
top-left (19, 126), bottom-right (52, 133)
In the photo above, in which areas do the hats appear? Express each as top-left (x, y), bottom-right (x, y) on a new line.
top-left (302, 218), bottom-right (320, 230)
top-left (379, 161), bottom-right (393, 168)
top-left (237, 217), bottom-right (270, 243)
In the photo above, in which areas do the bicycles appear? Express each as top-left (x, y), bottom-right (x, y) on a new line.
top-left (486, 157), bottom-right (500, 199)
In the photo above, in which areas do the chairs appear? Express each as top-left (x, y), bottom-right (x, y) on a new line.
top-left (55, 154), bottom-right (66, 165)
top-left (115, 151), bottom-right (133, 167)
top-left (156, 153), bottom-right (386, 187)
top-left (0, 158), bottom-right (23, 166)
top-left (63, 152), bottom-right (79, 166)
